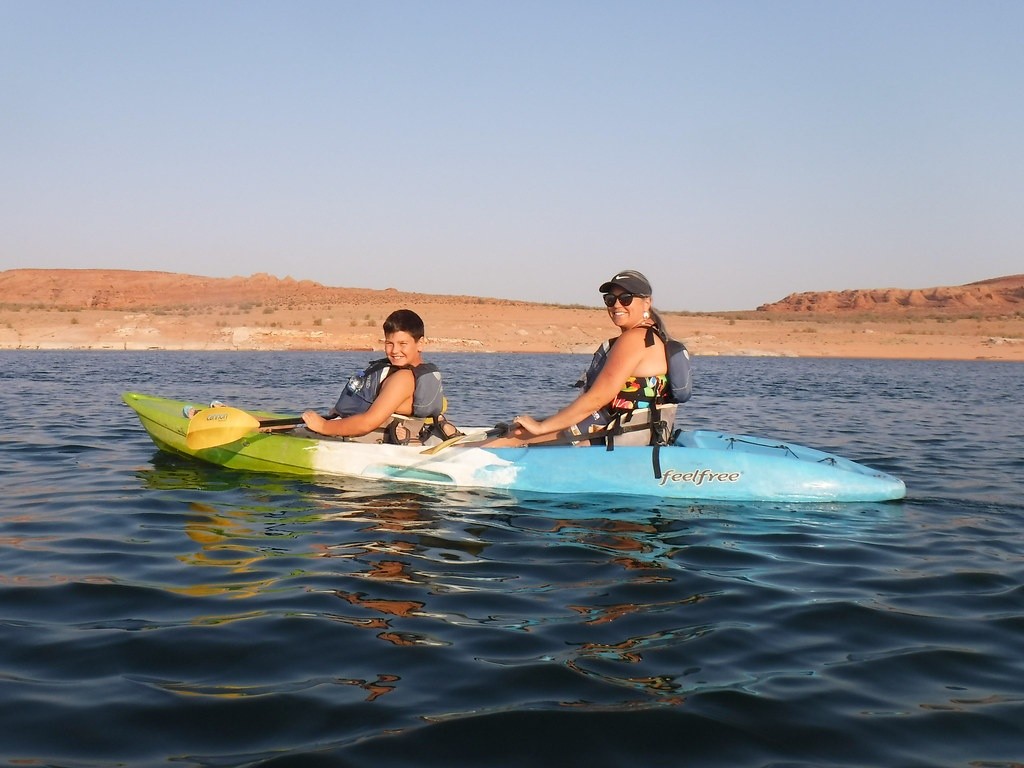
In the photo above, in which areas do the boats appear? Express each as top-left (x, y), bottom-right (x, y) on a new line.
top-left (120, 384), bottom-right (906, 510)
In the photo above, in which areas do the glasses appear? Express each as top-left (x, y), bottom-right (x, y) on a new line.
top-left (603, 292), bottom-right (647, 308)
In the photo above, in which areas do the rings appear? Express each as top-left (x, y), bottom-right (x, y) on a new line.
top-left (516, 415), bottom-right (519, 418)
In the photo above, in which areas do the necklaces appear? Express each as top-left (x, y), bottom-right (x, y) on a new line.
top-left (630, 319), bottom-right (643, 328)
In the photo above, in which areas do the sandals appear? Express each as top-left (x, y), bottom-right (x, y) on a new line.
top-left (389, 420), bottom-right (424, 448)
top-left (183, 404), bottom-right (201, 419)
top-left (209, 399), bottom-right (227, 408)
top-left (433, 413), bottom-right (467, 441)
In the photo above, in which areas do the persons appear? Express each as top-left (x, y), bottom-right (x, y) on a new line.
top-left (182, 308), bottom-right (425, 442)
top-left (460, 269), bottom-right (678, 447)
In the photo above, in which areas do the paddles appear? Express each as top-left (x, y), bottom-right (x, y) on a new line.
top-left (186, 404), bottom-right (339, 451)
top-left (421, 414), bottom-right (549, 456)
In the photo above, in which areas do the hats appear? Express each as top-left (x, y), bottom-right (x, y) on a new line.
top-left (598, 270), bottom-right (652, 298)
